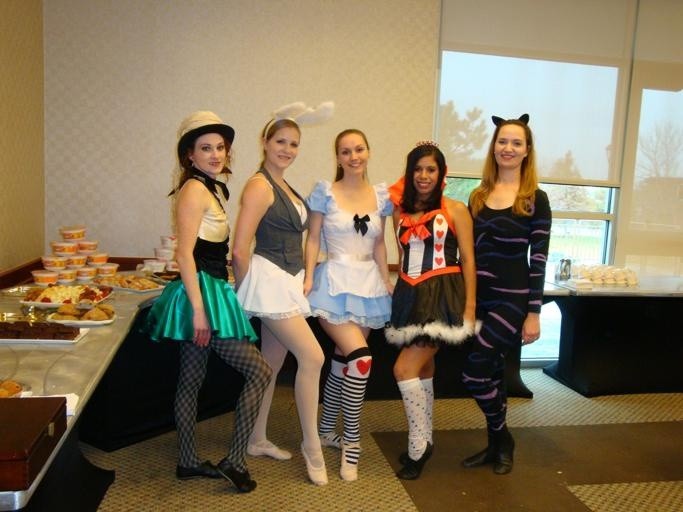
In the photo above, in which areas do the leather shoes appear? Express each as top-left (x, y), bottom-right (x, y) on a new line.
top-left (462, 443), bottom-right (495, 469)
top-left (493, 430), bottom-right (517, 475)
top-left (397, 442), bottom-right (433, 481)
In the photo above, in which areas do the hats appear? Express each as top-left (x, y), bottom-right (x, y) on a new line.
top-left (176, 109), bottom-right (235, 170)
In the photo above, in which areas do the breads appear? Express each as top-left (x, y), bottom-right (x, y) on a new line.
top-left (571, 262), bottom-right (638, 287)
top-left (1, 380), bottom-right (21, 398)
top-left (1, 322), bottom-right (80, 339)
top-left (52, 303), bottom-right (115, 321)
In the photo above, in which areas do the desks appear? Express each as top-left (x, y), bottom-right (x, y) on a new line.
top-left (2, 270), bottom-right (249, 455)
top-left (0, 304), bottom-right (142, 512)
top-left (542, 276), bottom-right (682, 401)
top-left (304, 281), bottom-right (570, 402)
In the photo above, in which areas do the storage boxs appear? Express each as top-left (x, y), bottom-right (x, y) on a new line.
top-left (0, 396), bottom-right (69, 491)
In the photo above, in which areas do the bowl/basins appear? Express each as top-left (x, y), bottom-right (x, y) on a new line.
top-left (143, 231), bottom-right (178, 273)
top-left (31, 226), bottom-right (120, 288)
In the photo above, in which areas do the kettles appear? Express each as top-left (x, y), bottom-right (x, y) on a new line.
top-left (553, 258), bottom-right (571, 283)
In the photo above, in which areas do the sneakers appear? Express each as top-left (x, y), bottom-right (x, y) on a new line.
top-left (247, 441), bottom-right (294, 461)
top-left (175, 459), bottom-right (222, 480)
top-left (215, 455), bottom-right (258, 494)
top-left (300, 439), bottom-right (329, 488)
top-left (340, 440), bottom-right (362, 482)
top-left (320, 433), bottom-right (364, 451)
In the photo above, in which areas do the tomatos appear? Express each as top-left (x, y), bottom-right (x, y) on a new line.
top-left (102, 290), bottom-right (109, 298)
top-left (64, 298), bottom-right (73, 304)
top-left (42, 298), bottom-right (51, 303)
top-left (95, 295), bottom-right (102, 301)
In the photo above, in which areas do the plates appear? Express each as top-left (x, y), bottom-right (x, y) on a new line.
top-left (0, 274), bottom-right (166, 345)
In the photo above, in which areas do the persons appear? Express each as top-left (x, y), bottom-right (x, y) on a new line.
top-left (384, 141), bottom-right (478, 480)
top-left (460, 112), bottom-right (552, 473)
top-left (304, 127), bottom-right (394, 481)
top-left (233, 116), bottom-right (329, 485)
top-left (138, 109), bottom-right (272, 493)
